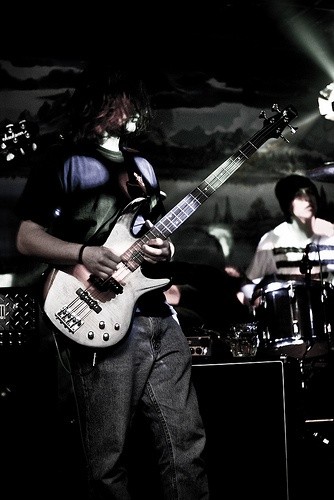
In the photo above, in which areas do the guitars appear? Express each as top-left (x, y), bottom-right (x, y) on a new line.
top-left (41, 104), bottom-right (300, 348)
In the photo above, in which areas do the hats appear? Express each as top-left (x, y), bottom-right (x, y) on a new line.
top-left (275, 175), bottom-right (322, 218)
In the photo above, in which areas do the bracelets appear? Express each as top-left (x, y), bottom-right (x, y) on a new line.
top-left (79, 243), bottom-right (86, 262)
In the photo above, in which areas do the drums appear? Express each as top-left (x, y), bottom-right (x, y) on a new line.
top-left (251, 279), bottom-right (333, 357)
top-left (226, 322), bottom-right (263, 358)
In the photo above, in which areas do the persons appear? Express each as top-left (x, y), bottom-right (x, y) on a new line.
top-left (247, 175), bottom-right (333, 302)
top-left (16, 67), bottom-right (210, 496)
